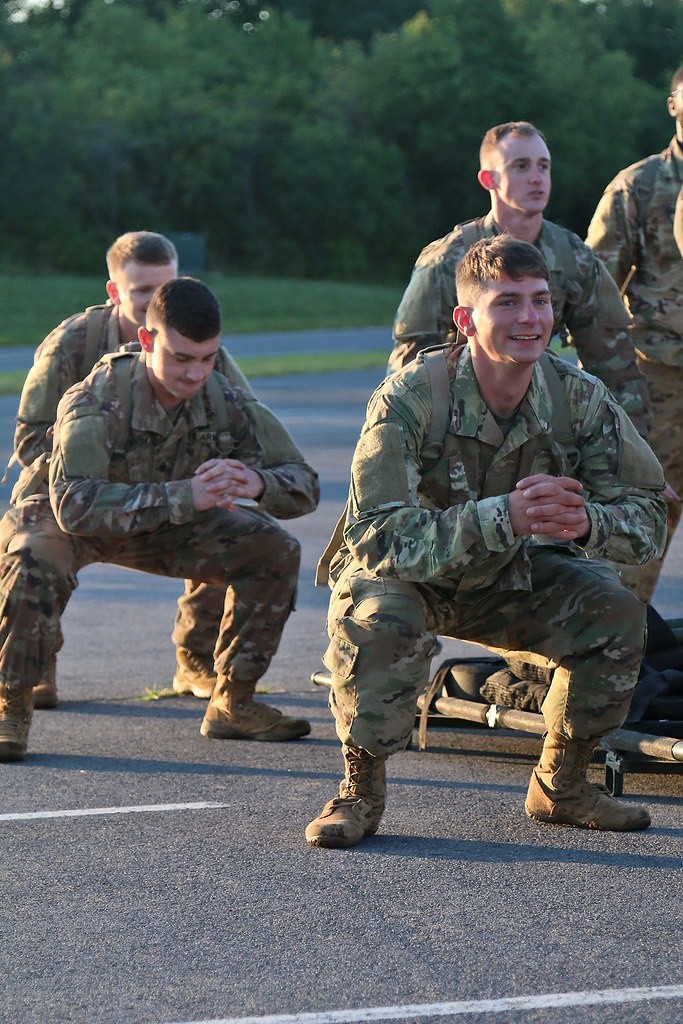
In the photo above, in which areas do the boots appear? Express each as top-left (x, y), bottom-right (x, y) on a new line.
top-left (32, 649), bottom-right (59, 711)
top-left (199, 672), bottom-right (311, 742)
top-left (304, 741), bottom-right (386, 849)
top-left (523, 730), bottom-right (652, 833)
top-left (0, 681), bottom-right (35, 760)
top-left (172, 647), bottom-right (218, 700)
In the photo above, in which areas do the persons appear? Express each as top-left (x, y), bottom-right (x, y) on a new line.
top-left (388, 122), bottom-right (678, 504)
top-left (12, 231), bottom-right (255, 708)
top-left (303, 236), bottom-right (669, 848)
top-left (579, 66), bottom-right (683, 607)
top-left (0, 277), bottom-right (320, 762)
